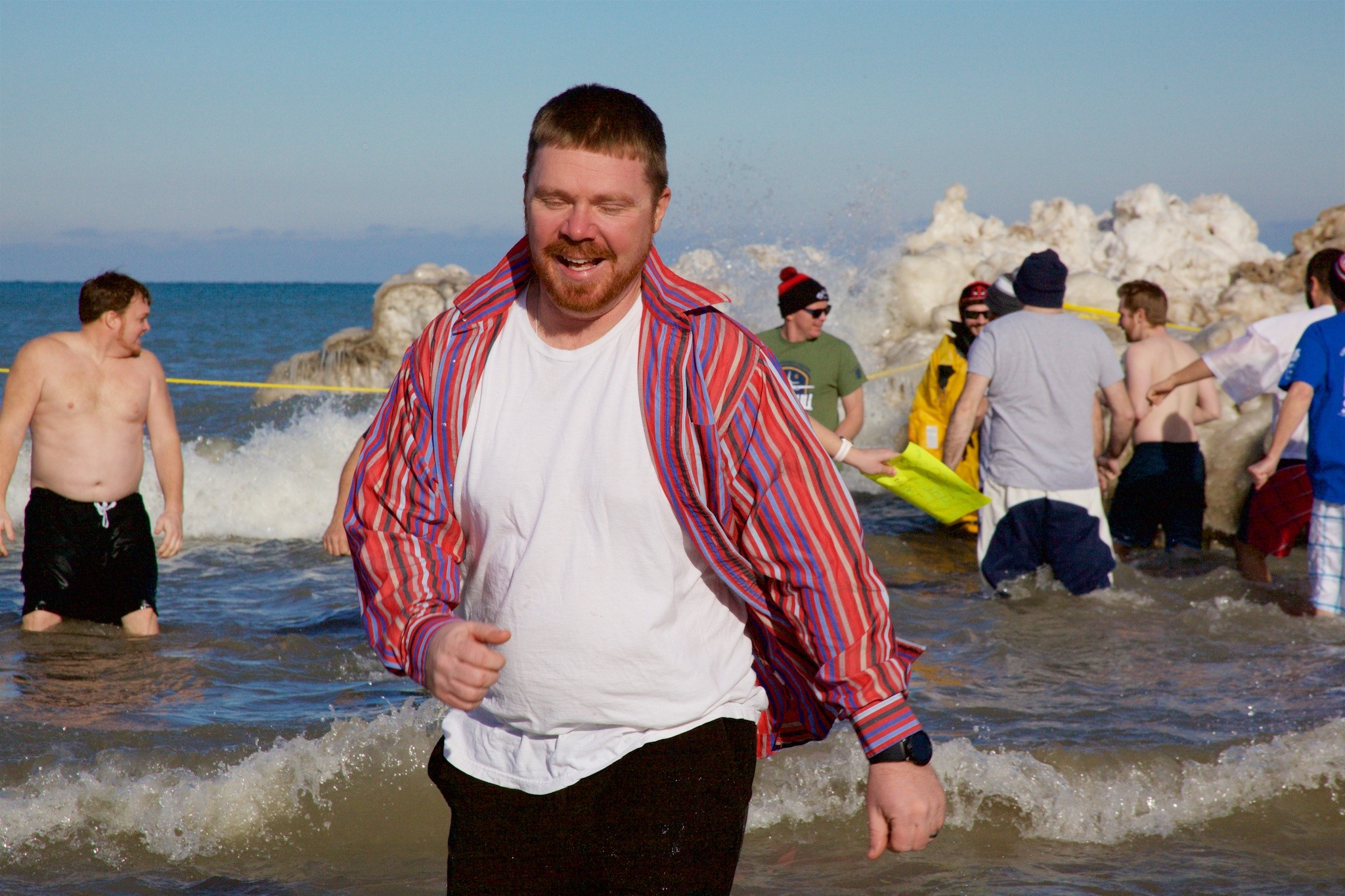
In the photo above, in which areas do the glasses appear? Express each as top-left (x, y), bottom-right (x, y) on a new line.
top-left (962, 309), bottom-right (992, 320)
top-left (804, 305), bottom-right (831, 318)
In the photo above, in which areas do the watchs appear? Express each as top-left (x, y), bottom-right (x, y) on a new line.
top-left (834, 435), bottom-right (854, 462)
top-left (869, 730), bottom-right (933, 767)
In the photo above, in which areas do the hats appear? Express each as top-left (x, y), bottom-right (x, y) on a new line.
top-left (958, 282), bottom-right (992, 317)
top-left (1330, 251), bottom-right (1345, 297)
top-left (778, 266), bottom-right (829, 317)
top-left (1013, 248), bottom-right (1067, 308)
top-left (985, 269), bottom-right (1020, 317)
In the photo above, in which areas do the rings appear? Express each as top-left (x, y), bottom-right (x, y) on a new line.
top-left (930, 830), bottom-right (938, 838)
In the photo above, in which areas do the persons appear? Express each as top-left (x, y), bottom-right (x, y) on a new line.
top-left (806, 412), bottom-right (901, 476)
top-left (1147, 246), bottom-right (1345, 587)
top-left (322, 426), bottom-right (372, 558)
top-left (1247, 252), bottom-right (1345, 618)
top-left (983, 267), bottom-right (1107, 496)
top-left (942, 248), bottom-right (1136, 603)
top-left (752, 266), bottom-right (869, 473)
top-left (0, 271), bottom-right (184, 636)
top-left (343, 85), bottom-right (946, 896)
top-left (1095, 280), bottom-right (1221, 561)
top-left (905, 281), bottom-right (989, 536)
